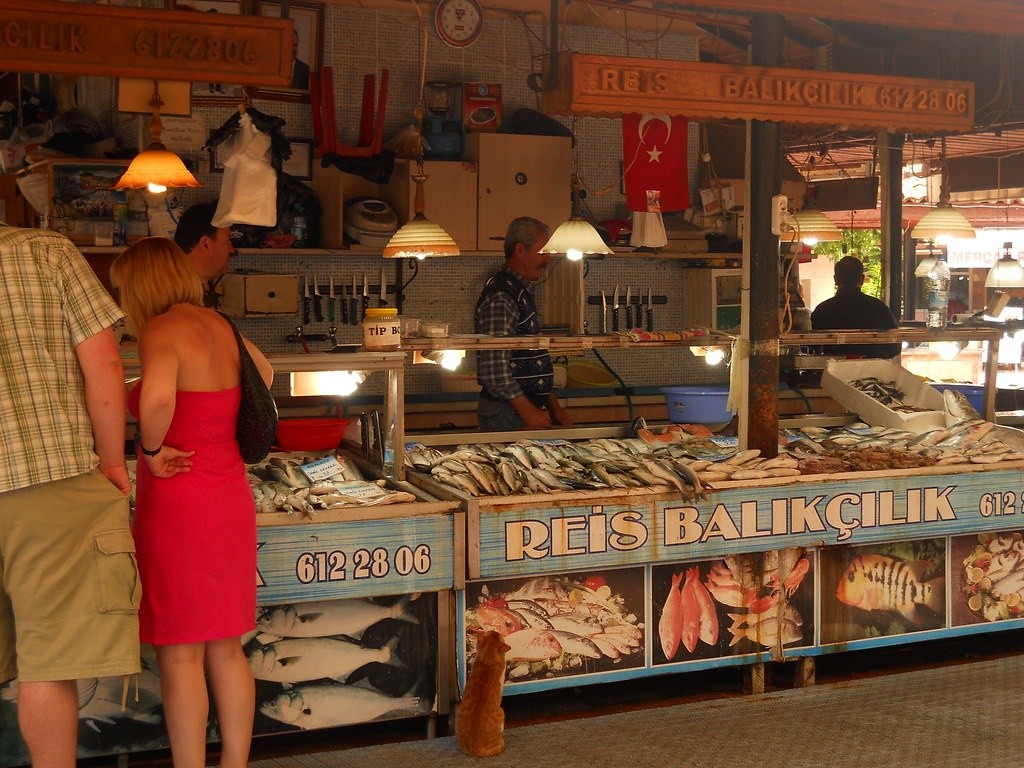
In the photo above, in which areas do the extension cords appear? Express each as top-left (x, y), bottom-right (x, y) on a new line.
top-left (772, 195), bottom-right (787, 235)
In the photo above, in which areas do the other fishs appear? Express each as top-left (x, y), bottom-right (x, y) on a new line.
top-left (258, 681), bottom-right (423, 730)
top-left (243, 626), bottom-right (408, 690)
top-left (779, 388), bottom-right (1024, 463)
top-left (681, 566), bottom-right (719, 653)
top-left (658, 570), bottom-right (683, 660)
top-left (257, 598), bottom-right (420, 638)
top-left (466, 579), bottom-right (642, 661)
top-left (408, 440), bottom-right (802, 505)
top-left (704, 548), bottom-right (805, 647)
top-left (835, 553), bottom-right (945, 627)
top-left (245, 456), bottom-right (417, 513)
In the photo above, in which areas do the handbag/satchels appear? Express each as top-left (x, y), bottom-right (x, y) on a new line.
top-left (217, 309), bottom-right (278, 464)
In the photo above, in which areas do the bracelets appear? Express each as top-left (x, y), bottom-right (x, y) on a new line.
top-left (140, 441), bottom-right (162, 457)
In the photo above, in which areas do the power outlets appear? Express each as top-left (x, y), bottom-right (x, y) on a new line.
top-left (772, 196), bottom-right (790, 234)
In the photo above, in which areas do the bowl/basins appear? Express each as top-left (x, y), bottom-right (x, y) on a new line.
top-left (398, 314), bottom-right (451, 339)
top-left (932, 385), bottom-right (999, 416)
top-left (660, 387), bottom-right (733, 424)
top-left (565, 362), bottom-right (617, 388)
top-left (276, 418), bottom-right (352, 451)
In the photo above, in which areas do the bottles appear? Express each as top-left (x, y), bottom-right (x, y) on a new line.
top-left (926, 255), bottom-right (951, 329)
top-left (363, 308), bottom-right (401, 352)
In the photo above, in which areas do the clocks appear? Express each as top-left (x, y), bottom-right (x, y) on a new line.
top-left (433, 0), bottom-right (484, 50)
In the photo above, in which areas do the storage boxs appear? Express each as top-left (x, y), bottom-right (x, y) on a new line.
top-left (821, 355), bottom-right (952, 435)
top-left (218, 270), bottom-right (301, 318)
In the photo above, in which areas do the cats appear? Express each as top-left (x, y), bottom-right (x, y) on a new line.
top-left (455, 630), bottom-right (511, 757)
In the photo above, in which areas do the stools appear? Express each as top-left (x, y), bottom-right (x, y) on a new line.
top-left (306, 65), bottom-right (390, 158)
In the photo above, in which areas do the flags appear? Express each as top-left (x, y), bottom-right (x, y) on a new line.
top-left (622, 112), bottom-right (689, 212)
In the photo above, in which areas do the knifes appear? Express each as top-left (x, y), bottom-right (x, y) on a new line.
top-left (353, 409), bottom-right (383, 469)
top-left (647, 288), bottom-right (654, 332)
top-left (637, 287), bottom-right (643, 328)
top-left (613, 285), bottom-right (619, 331)
top-left (602, 291), bottom-right (607, 333)
top-left (625, 287), bottom-right (633, 329)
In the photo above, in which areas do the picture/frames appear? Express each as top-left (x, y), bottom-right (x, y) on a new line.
top-left (243, 0), bottom-right (329, 103)
top-left (209, 128), bottom-right (231, 172)
top-left (275, 137), bottom-right (314, 181)
top-left (172, 0), bottom-right (253, 108)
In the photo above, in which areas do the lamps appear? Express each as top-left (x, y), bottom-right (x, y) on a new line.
top-left (914, 242), bottom-right (950, 277)
top-left (383, 7), bottom-right (461, 260)
top-left (781, 151), bottom-right (843, 244)
top-left (983, 133), bottom-right (1023, 288)
top-left (537, 115), bottom-right (614, 263)
top-left (911, 128), bottom-right (976, 239)
top-left (117, 85), bottom-right (203, 194)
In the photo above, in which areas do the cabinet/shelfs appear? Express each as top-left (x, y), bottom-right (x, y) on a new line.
top-left (683, 269), bottom-right (746, 331)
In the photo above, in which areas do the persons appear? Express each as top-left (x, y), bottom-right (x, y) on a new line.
top-left (474, 217), bottom-right (572, 429)
top-left (0, 222), bottom-right (134, 768)
top-left (136, 204), bottom-right (235, 454)
top-left (110, 237), bottom-right (274, 768)
top-left (811, 256), bottom-right (902, 361)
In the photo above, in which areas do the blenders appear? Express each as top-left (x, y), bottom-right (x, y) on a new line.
top-left (422, 79), bottom-right (466, 161)
